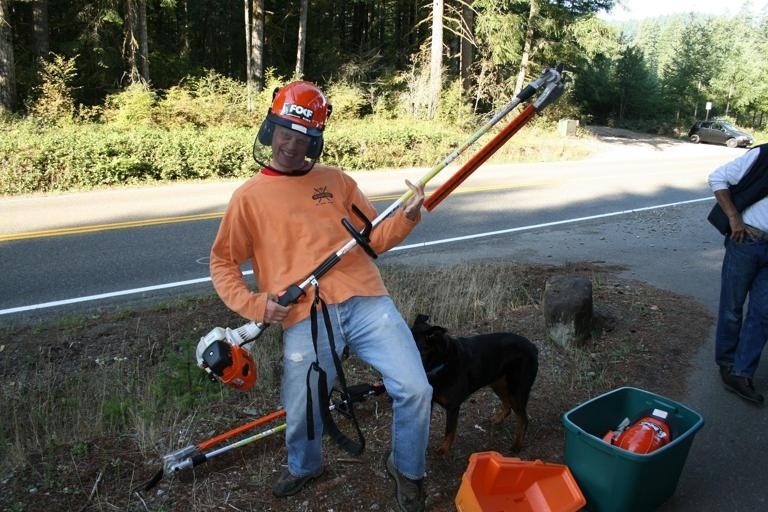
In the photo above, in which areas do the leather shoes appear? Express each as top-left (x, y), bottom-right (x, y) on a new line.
top-left (719, 365), bottom-right (735, 391)
top-left (723, 376), bottom-right (764, 404)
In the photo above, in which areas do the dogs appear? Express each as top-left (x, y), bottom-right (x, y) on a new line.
top-left (409, 312), bottom-right (540, 456)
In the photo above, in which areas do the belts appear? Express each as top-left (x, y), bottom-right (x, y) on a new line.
top-left (745, 224), bottom-right (767, 241)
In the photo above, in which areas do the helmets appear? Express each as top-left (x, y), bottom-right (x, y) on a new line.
top-left (615, 413), bottom-right (671, 455)
top-left (253, 81), bottom-right (333, 176)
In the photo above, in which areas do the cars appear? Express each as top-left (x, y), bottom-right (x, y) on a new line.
top-left (688, 119), bottom-right (753, 148)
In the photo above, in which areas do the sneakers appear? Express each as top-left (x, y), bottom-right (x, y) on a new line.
top-left (385, 452), bottom-right (426, 512)
top-left (272, 464), bottom-right (325, 497)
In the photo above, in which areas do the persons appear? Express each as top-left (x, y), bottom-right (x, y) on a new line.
top-left (210, 81), bottom-right (436, 512)
top-left (705, 144), bottom-right (767, 405)
